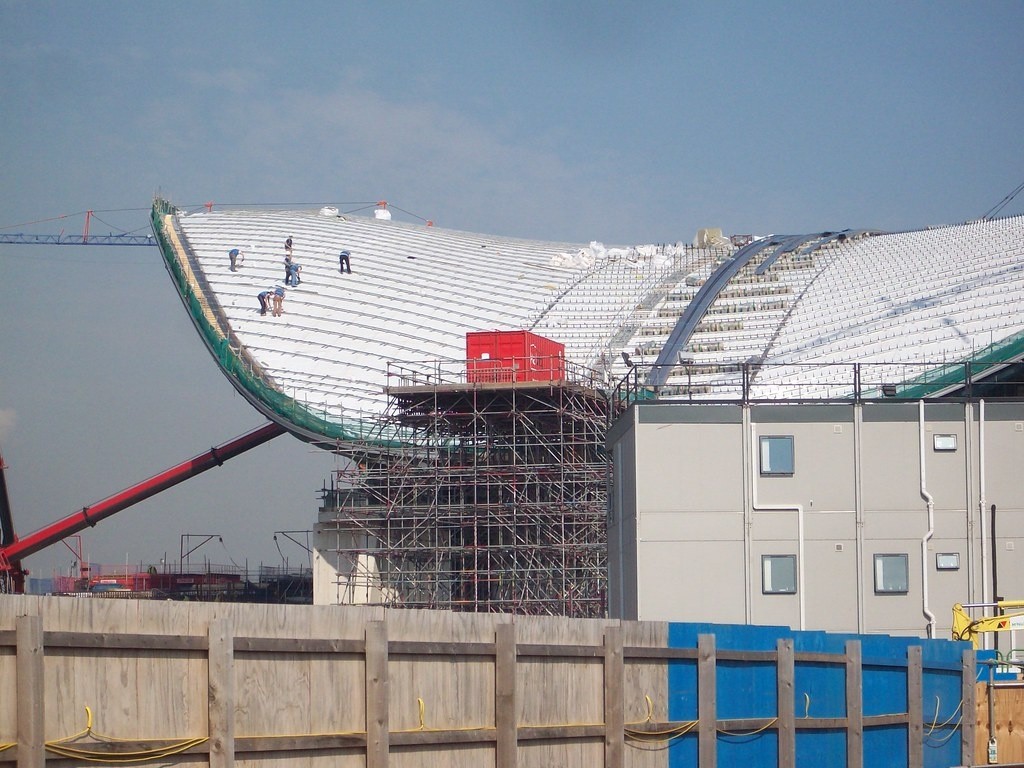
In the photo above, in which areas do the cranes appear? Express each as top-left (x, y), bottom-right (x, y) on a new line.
top-left (0, 193), bottom-right (437, 250)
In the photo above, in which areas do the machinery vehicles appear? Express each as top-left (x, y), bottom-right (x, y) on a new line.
top-left (0, 420), bottom-right (292, 595)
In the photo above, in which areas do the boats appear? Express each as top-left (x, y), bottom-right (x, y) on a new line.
top-left (50, 532), bottom-right (270, 601)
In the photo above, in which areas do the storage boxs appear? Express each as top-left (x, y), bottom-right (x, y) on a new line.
top-left (466, 331), bottom-right (565, 383)
top-left (28, 573), bottom-right (240, 596)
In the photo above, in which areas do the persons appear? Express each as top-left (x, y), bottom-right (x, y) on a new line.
top-left (180, 592), bottom-right (190, 600)
top-left (258, 290), bottom-right (273, 315)
top-left (284, 254), bottom-right (303, 287)
top-left (147, 565), bottom-right (158, 575)
top-left (285, 238), bottom-right (293, 252)
top-left (272, 287), bottom-right (285, 317)
top-left (340, 250), bottom-right (352, 274)
top-left (228, 248), bottom-right (245, 273)
